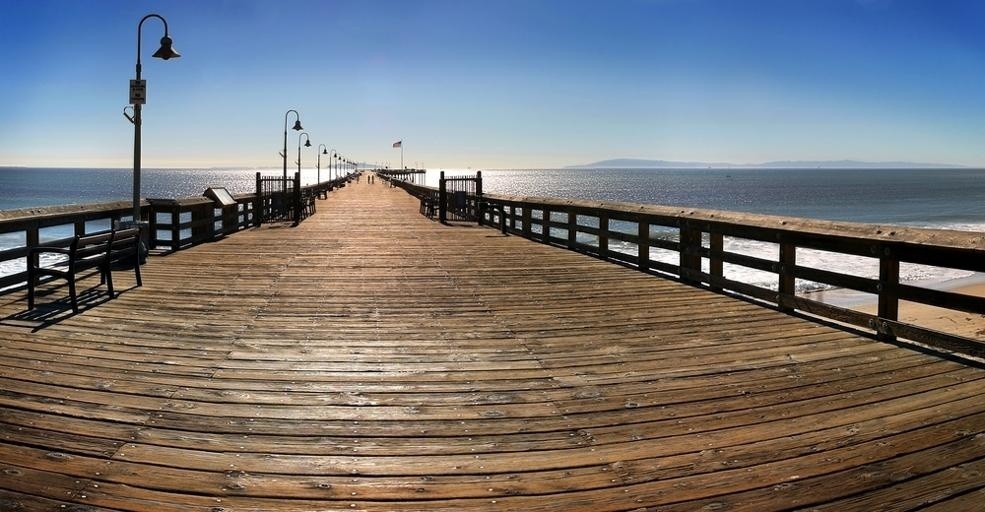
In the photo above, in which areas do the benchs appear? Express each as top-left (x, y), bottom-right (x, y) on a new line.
top-left (315, 174), bottom-right (352, 199)
top-left (23, 222), bottom-right (144, 313)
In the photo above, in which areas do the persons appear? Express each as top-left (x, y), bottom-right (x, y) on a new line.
top-left (356, 173), bottom-right (374, 184)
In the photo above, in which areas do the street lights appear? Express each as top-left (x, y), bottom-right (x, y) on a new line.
top-left (281, 109), bottom-right (358, 194)
top-left (131, 15), bottom-right (181, 242)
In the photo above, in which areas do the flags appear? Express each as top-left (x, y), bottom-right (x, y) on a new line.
top-left (393, 141), bottom-right (401, 147)
